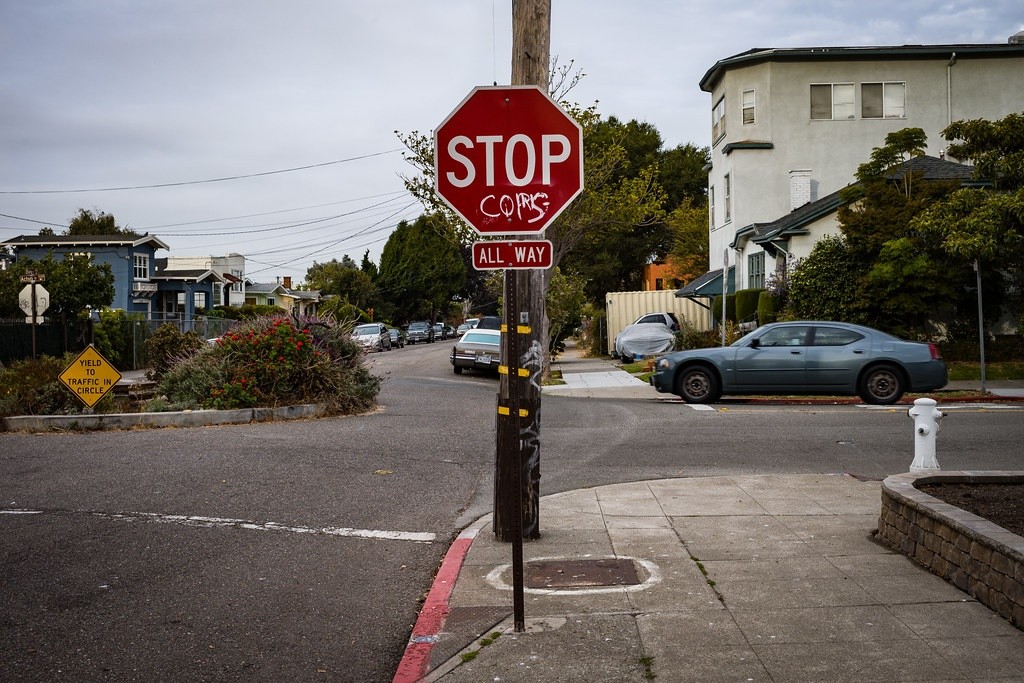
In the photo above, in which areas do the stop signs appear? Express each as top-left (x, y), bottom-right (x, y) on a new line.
top-left (433, 84), bottom-right (584, 237)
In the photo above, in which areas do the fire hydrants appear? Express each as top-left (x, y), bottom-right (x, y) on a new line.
top-left (905, 397), bottom-right (949, 476)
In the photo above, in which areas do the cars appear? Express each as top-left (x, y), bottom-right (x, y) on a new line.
top-left (433, 321), bottom-right (456, 341)
top-left (615, 312), bottom-right (682, 364)
top-left (457, 325), bottom-right (473, 337)
top-left (449, 328), bottom-right (502, 374)
top-left (650, 320), bottom-right (951, 411)
top-left (389, 329), bottom-right (405, 349)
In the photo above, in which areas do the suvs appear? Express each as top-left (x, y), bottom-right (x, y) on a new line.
top-left (405, 321), bottom-right (435, 345)
top-left (477, 316), bottom-right (503, 332)
top-left (349, 322), bottom-right (392, 353)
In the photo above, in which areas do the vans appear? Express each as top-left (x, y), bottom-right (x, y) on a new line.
top-left (465, 318), bottom-right (480, 328)
